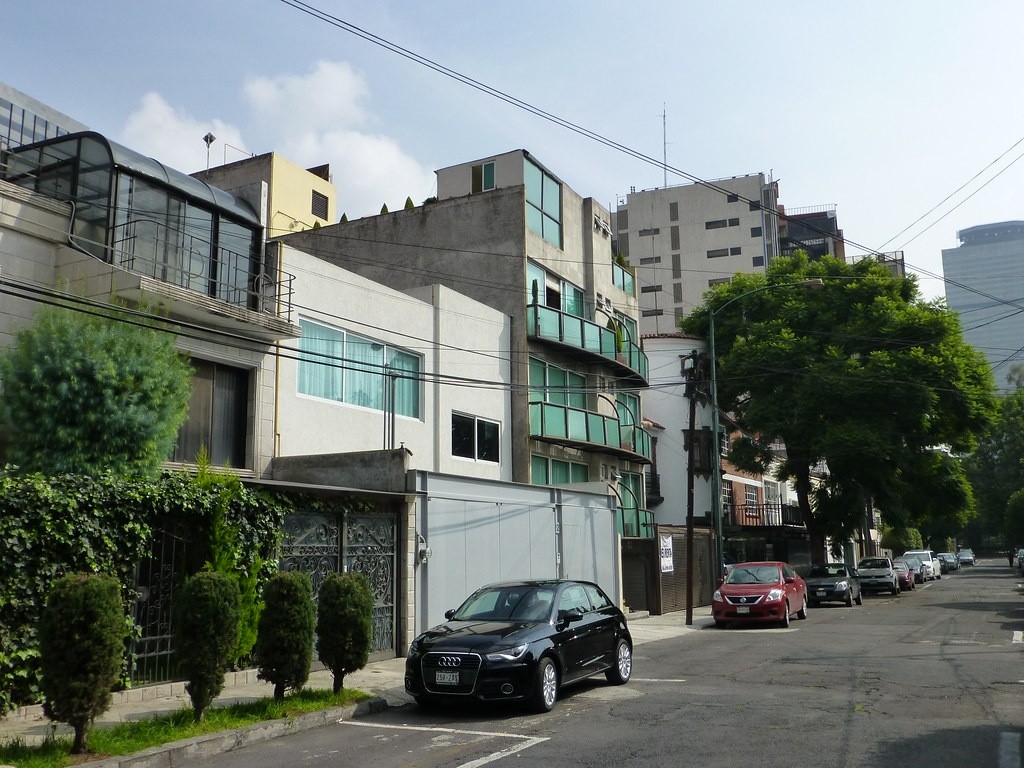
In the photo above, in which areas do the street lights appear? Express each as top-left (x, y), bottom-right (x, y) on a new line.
top-left (710, 278), bottom-right (823, 589)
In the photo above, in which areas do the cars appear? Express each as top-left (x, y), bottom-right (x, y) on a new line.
top-left (404, 580), bottom-right (633, 712)
top-left (713, 562), bottom-right (807, 628)
top-left (1015, 549), bottom-right (1024, 569)
top-left (805, 563), bottom-right (863, 607)
top-left (891, 551), bottom-right (976, 591)
top-left (857, 556), bottom-right (901, 596)
